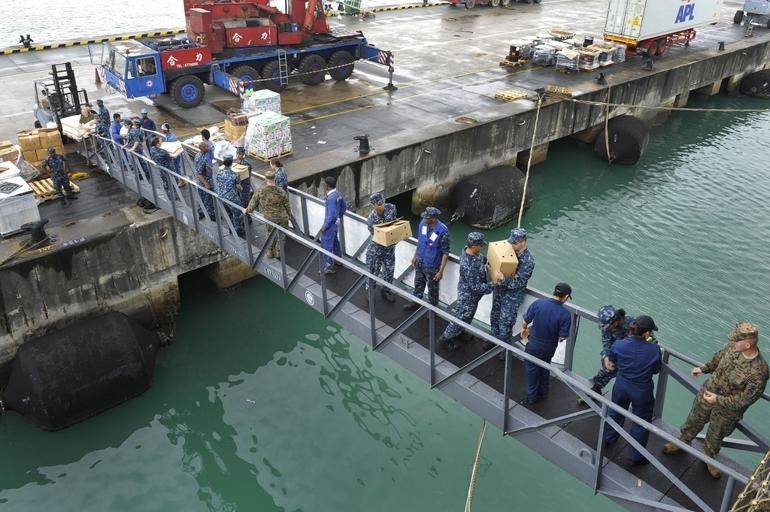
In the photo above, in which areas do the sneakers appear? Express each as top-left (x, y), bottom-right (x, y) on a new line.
top-left (381, 287), bottom-right (396, 304)
top-left (496, 348), bottom-right (507, 362)
top-left (622, 455), bottom-right (649, 467)
top-left (603, 433), bottom-right (622, 451)
top-left (481, 339), bottom-right (498, 351)
top-left (161, 177), bottom-right (187, 201)
top-left (402, 302), bottom-right (421, 312)
top-left (576, 391), bottom-right (602, 407)
top-left (520, 393), bottom-right (549, 407)
top-left (364, 292), bottom-right (376, 307)
top-left (335, 260), bottom-right (343, 266)
top-left (318, 266), bottom-right (337, 275)
top-left (123, 165), bottom-right (151, 184)
top-left (198, 211), bottom-right (252, 238)
top-left (436, 337), bottom-right (461, 352)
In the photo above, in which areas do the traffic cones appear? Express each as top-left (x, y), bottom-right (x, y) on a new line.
top-left (95, 65), bottom-right (102, 83)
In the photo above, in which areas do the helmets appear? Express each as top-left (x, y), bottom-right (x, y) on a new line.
top-left (140, 108), bottom-right (148, 114)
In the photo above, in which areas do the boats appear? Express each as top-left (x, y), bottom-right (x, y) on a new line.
top-left (738, 67), bottom-right (770, 100)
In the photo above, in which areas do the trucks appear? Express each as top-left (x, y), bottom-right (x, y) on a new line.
top-left (443, 0), bottom-right (542, 8)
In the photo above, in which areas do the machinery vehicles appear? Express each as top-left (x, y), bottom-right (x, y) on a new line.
top-left (98, 0), bottom-right (400, 112)
top-left (30, 62), bottom-right (99, 146)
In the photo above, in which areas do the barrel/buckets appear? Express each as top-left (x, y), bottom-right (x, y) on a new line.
top-left (343, 0), bottom-right (362, 12)
top-left (509, 44), bottom-right (520, 62)
top-left (583, 35), bottom-right (594, 48)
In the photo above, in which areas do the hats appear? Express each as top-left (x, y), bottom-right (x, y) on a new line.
top-left (264, 170), bottom-right (276, 180)
top-left (420, 206), bottom-right (442, 220)
top-left (223, 154), bottom-right (234, 163)
top-left (554, 282), bottom-right (573, 301)
top-left (632, 314), bottom-right (659, 334)
top-left (96, 99), bottom-right (104, 105)
top-left (725, 320), bottom-right (760, 343)
top-left (47, 146), bottom-right (55, 155)
top-left (597, 303), bottom-right (621, 332)
top-left (122, 113), bottom-right (141, 127)
top-left (368, 192), bottom-right (386, 206)
top-left (466, 230), bottom-right (489, 247)
top-left (507, 227), bottom-right (527, 246)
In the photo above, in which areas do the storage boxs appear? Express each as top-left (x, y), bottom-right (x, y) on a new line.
top-left (372, 215), bottom-right (414, 247)
top-left (0, 175), bottom-right (41, 237)
top-left (217, 162), bottom-right (249, 183)
top-left (512, 30), bottom-right (628, 73)
top-left (486, 237), bottom-right (519, 284)
top-left (223, 116), bottom-right (245, 144)
top-left (0, 124), bottom-right (68, 180)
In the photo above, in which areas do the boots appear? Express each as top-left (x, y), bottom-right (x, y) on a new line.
top-left (698, 447), bottom-right (722, 480)
top-left (662, 436), bottom-right (693, 457)
top-left (60, 196), bottom-right (67, 206)
top-left (67, 193), bottom-right (79, 200)
top-left (266, 247), bottom-right (281, 259)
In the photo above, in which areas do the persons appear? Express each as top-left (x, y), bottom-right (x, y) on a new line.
top-left (41, 146), bottom-right (74, 206)
top-left (436, 232), bottom-right (503, 353)
top-left (366, 193), bottom-right (398, 303)
top-left (404, 207), bottom-right (450, 320)
top-left (577, 305), bottom-right (658, 404)
top-left (663, 323), bottom-right (770, 478)
top-left (78, 100), bottom-right (292, 260)
top-left (604, 316), bottom-right (663, 464)
top-left (520, 283), bottom-right (572, 404)
top-left (482, 227), bottom-right (535, 361)
top-left (317, 177), bottom-right (347, 275)
